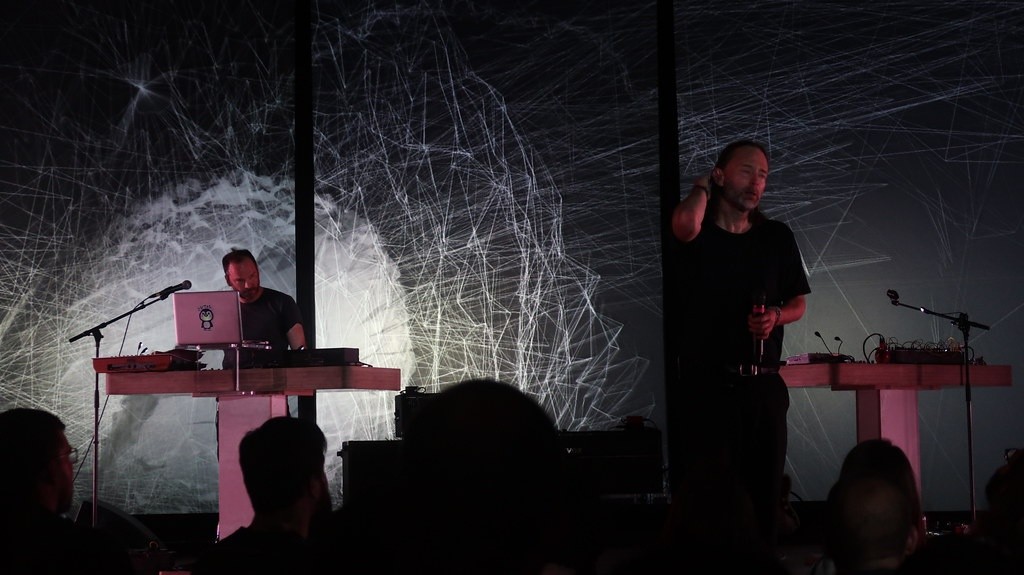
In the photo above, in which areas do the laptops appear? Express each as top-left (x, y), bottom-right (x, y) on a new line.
top-left (173, 290), bottom-right (270, 344)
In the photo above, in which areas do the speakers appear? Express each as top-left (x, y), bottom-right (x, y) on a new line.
top-left (75, 497), bottom-right (165, 550)
top-left (395, 393), bottom-right (436, 438)
top-left (560, 429), bottom-right (663, 495)
top-left (340, 440), bottom-right (403, 495)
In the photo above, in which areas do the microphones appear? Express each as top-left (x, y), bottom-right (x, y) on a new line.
top-left (149, 280), bottom-right (192, 298)
top-left (752, 294), bottom-right (767, 362)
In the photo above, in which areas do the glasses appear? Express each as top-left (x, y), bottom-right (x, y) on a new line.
top-left (49, 448), bottom-right (78, 464)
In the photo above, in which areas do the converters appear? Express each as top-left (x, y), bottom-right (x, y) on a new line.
top-left (624, 416), bottom-right (645, 430)
top-left (406, 386), bottom-right (419, 394)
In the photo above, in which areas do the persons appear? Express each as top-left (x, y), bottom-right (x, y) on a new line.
top-left (192, 378), bottom-right (1024, 575)
top-left (0, 408), bottom-right (133, 575)
top-left (176, 249), bottom-right (308, 349)
top-left (671, 139), bottom-right (812, 531)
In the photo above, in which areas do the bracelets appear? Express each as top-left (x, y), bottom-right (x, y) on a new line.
top-left (691, 184), bottom-right (709, 198)
top-left (770, 306), bottom-right (781, 326)
